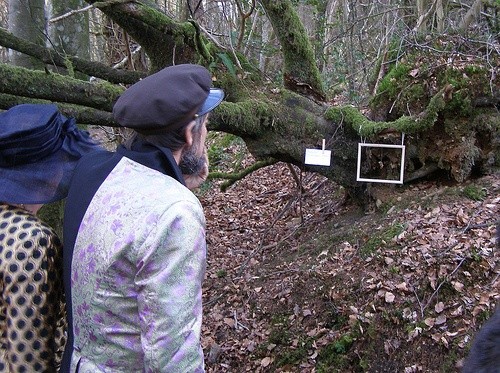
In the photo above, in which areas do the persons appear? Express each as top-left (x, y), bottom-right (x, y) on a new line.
top-left (0, 103), bottom-right (108, 373)
top-left (61, 63), bottom-right (225, 373)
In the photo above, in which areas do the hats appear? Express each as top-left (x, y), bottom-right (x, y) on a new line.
top-left (1, 103), bottom-right (109, 205)
top-left (111, 64), bottom-right (225, 131)
